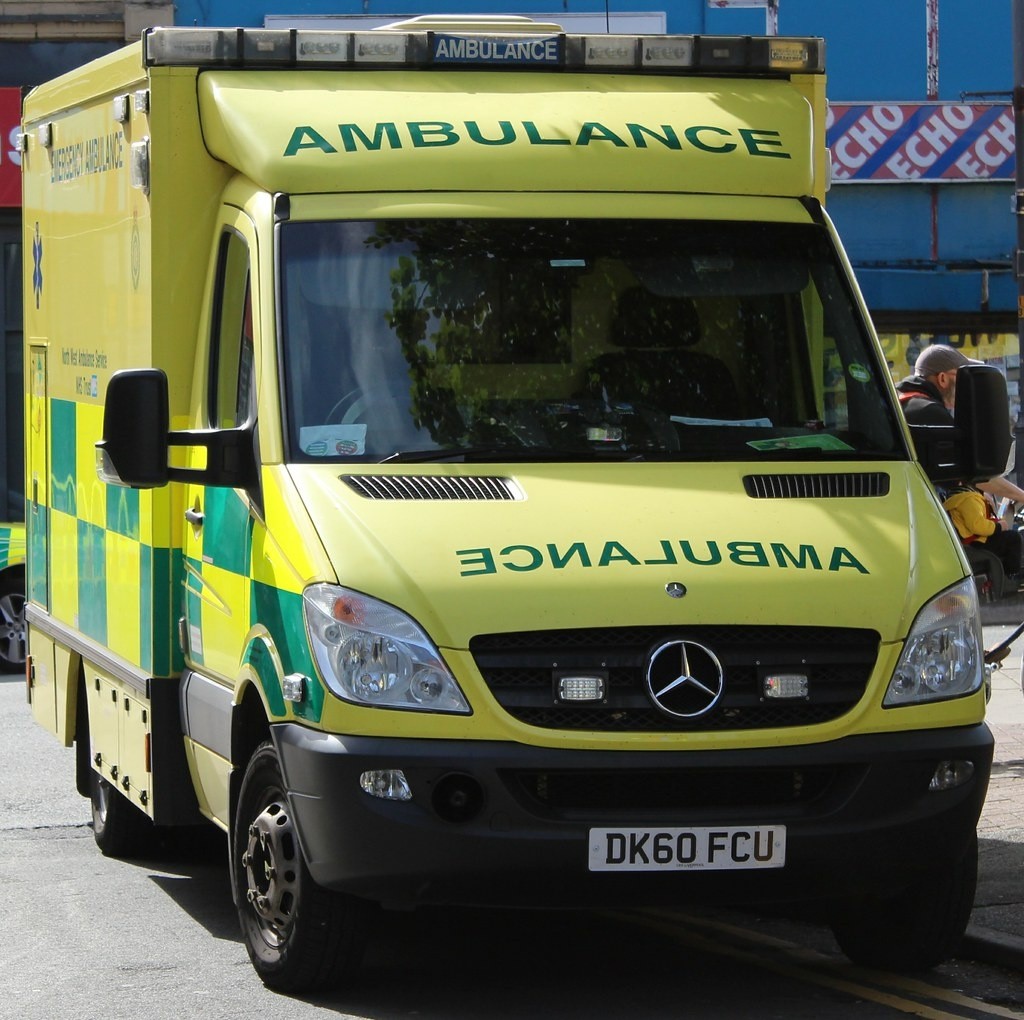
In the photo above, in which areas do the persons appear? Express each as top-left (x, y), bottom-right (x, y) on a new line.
top-left (941, 478), bottom-right (1024, 592)
top-left (895, 344), bottom-right (1024, 505)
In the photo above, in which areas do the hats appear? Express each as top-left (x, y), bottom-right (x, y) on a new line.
top-left (915, 345), bottom-right (986, 377)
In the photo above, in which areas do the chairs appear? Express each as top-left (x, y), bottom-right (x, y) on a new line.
top-left (573, 287), bottom-right (749, 459)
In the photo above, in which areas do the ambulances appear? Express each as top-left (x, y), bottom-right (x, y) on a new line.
top-left (16, 12), bottom-right (1024, 999)
